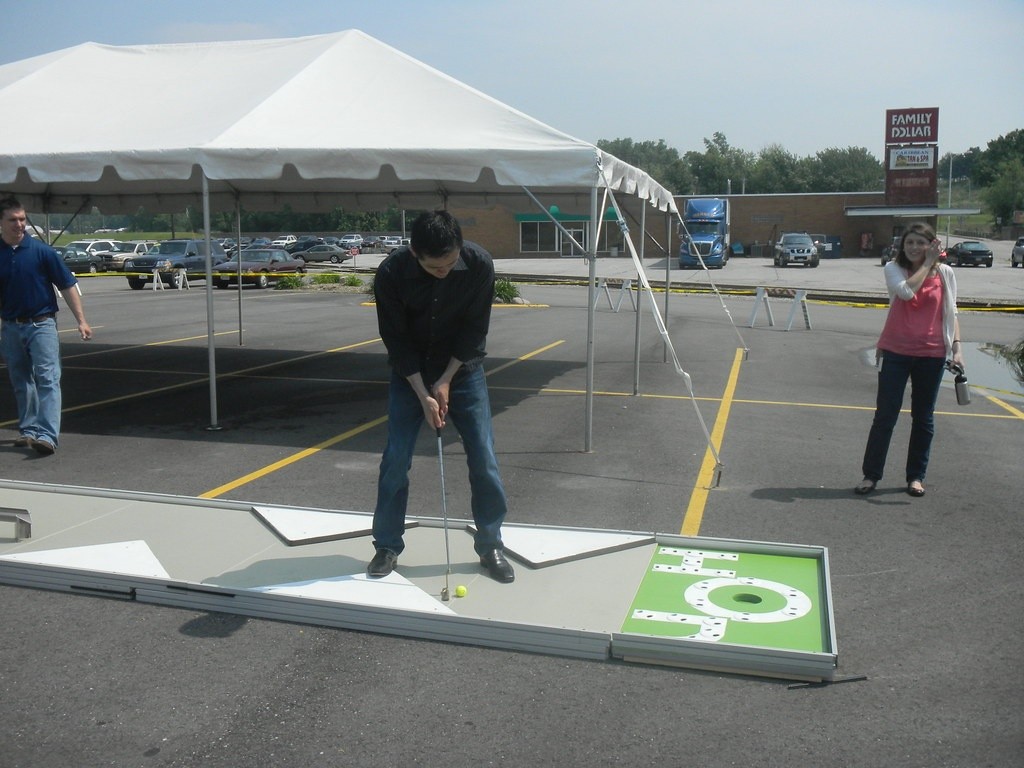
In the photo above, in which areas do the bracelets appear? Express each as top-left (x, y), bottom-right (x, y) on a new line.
top-left (953, 340), bottom-right (960, 343)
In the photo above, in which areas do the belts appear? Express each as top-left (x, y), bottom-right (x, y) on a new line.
top-left (1, 313), bottom-right (57, 324)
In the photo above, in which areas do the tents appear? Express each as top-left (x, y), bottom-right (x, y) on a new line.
top-left (0, 29), bottom-right (676, 453)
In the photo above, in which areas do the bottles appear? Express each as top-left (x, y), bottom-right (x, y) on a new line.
top-left (954, 375), bottom-right (971, 405)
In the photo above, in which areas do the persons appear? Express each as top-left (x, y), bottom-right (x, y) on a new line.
top-left (853, 222), bottom-right (965, 499)
top-left (0, 196), bottom-right (94, 453)
top-left (366, 208), bottom-right (516, 583)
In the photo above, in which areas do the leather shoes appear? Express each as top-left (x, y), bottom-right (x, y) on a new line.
top-left (367, 547), bottom-right (397, 575)
top-left (480, 549), bottom-right (515, 584)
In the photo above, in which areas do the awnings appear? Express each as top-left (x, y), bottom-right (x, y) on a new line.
top-left (514, 205), bottom-right (620, 222)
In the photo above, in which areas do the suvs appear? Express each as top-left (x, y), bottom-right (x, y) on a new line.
top-left (123, 237), bottom-right (228, 290)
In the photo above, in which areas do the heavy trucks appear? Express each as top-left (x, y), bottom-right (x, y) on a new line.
top-left (676, 198), bottom-right (731, 270)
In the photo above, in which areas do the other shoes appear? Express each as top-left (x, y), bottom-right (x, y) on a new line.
top-left (16, 436), bottom-right (32, 446)
top-left (854, 477), bottom-right (876, 494)
top-left (32, 441), bottom-right (53, 453)
top-left (907, 481), bottom-right (924, 496)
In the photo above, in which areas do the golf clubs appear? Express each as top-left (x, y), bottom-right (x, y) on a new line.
top-left (436, 426), bottom-right (451, 601)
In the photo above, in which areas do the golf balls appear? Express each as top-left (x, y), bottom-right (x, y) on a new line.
top-left (456, 586), bottom-right (466, 597)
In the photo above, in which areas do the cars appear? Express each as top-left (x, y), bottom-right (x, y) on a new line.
top-left (211, 248), bottom-right (308, 290)
top-left (50, 246), bottom-right (107, 278)
top-left (880, 236), bottom-right (904, 266)
top-left (773, 232), bottom-right (820, 268)
top-left (45, 232), bottom-right (412, 278)
top-left (291, 244), bottom-right (354, 264)
top-left (1011, 235), bottom-right (1024, 269)
top-left (944, 240), bottom-right (993, 268)
